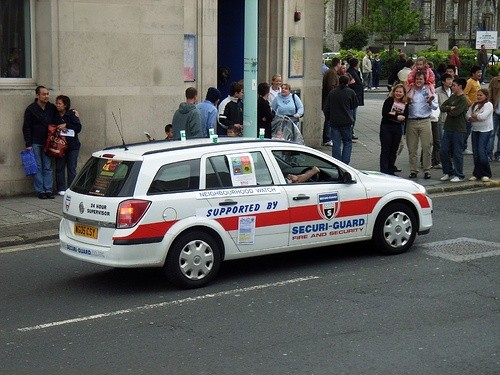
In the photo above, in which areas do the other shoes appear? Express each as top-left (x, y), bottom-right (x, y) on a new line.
top-left (440, 172), bottom-right (455, 180)
top-left (424, 172), bottom-right (432, 179)
top-left (450, 176), bottom-right (464, 182)
top-left (468, 176), bottom-right (477, 180)
top-left (394, 166), bottom-right (402, 172)
top-left (408, 172), bottom-right (417, 178)
top-left (352, 133), bottom-right (358, 139)
top-left (46, 192), bottom-right (55, 199)
top-left (481, 176), bottom-right (490, 182)
top-left (324, 141), bottom-right (333, 146)
top-left (39, 193), bottom-right (47, 199)
top-left (57, 191), bottom-right (65, 196)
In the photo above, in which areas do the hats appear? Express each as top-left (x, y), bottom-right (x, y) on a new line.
top-left (206, 87), bottom-right (222, 103)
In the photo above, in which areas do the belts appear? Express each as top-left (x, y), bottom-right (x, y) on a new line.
top-left (409, 117), bottom-right (430, 120)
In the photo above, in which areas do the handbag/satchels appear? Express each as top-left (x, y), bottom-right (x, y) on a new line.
top-left (20, 150), bottom-right (39, 176)
top-left (45, 129), bottom-right (67, 158)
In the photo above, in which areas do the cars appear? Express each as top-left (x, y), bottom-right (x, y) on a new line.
top-left (473, 53), bottom-right (500, 67)
top-left (322, 52), bottom-right (341, 62)
top-left (55, 136), bottom-right (436, 290)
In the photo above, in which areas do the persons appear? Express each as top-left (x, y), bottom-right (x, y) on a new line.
top-left (172, 87), bottom-right (203, 138)
top-left (380, 52), bottom-right (500, 182)
top-left (362, 50), bottom-right (381, 91)
top-left (218, 74), bottom-right (304, 139)
top-left (477, 44), bottom-right (488, 85)
top-left (52, 95), bottom-right (82, 196)
top-left (22, 86), bottom-right (79, 200)
top-left (285, 166), bottom-right (319, 183)
top-left (321, 55), bottom-right (364, 147)
top-left (196, 87), bottom-right (221, 138)
top-left (450, 46), bottom-right (461, 75)
top-left (323, 76), bottom-right (359, 165)
top-left (165, 124), bottom-right (173, 140)
